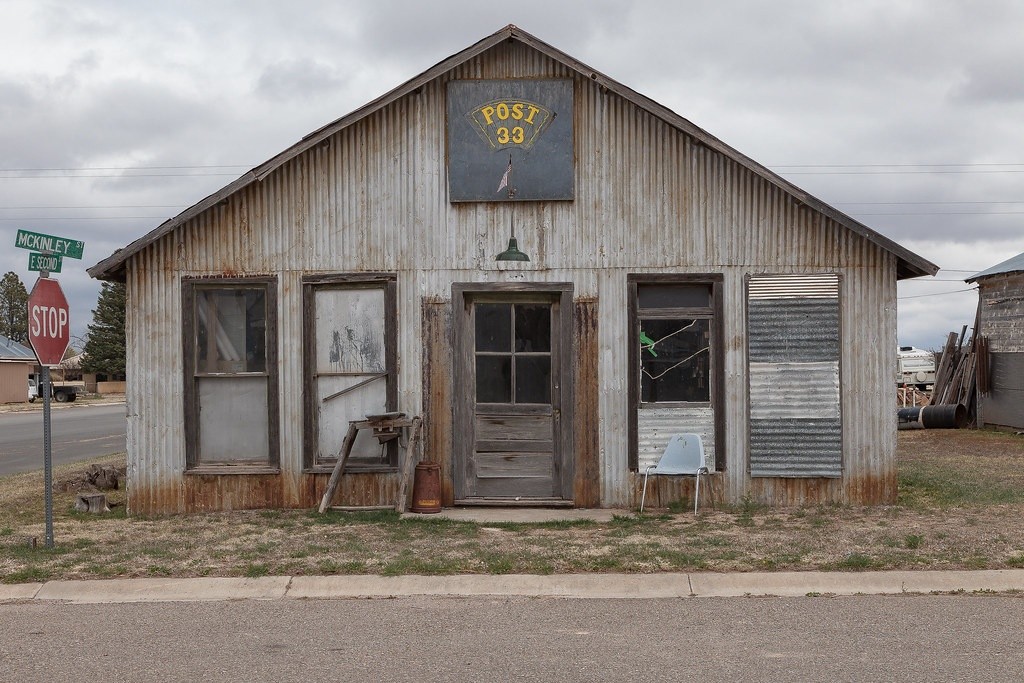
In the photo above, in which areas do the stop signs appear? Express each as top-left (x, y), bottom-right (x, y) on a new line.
top-left (26, 278), bottom-right (71, 366)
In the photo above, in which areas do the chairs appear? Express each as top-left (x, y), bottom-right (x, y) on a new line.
top-left (641, 433), bottom-right (716, 516)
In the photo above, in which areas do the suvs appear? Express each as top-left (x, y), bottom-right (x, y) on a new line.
top-left (28, 379), bottom-right (38, 402)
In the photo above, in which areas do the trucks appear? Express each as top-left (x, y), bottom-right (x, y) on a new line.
top-left (28, 373), bottom-right (84, 402)
top-left (896, 346), bottom-right (935, 391)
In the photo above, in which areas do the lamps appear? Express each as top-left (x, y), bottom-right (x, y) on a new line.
top-left (495, 188), bottom-right (530, 261)
